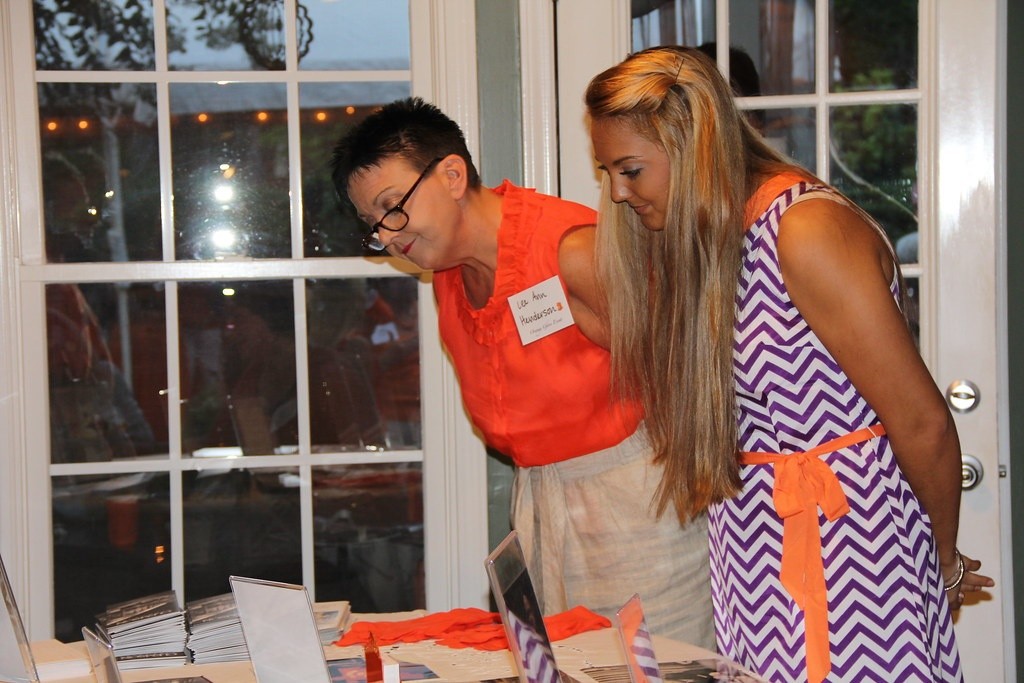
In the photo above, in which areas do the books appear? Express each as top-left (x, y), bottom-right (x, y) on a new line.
top-left (98, 577), bottom-right (352, 672)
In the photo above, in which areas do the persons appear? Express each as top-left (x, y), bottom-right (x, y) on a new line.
top-left (22, 124), bottom-right (434, 642)
top-left (333, 93), bottom-right (722, 659)
top-left (583, 42), bottom-right (998, 683)
top-left (522, 587), bottom-right (543, 631)
top-left (693, 42), bottom-right (766, 139)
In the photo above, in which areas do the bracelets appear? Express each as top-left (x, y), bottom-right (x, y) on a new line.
top-left (943, 545), bottom-right (964, 592)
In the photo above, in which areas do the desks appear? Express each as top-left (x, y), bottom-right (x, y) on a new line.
top-left (28, 608), bottom-right (769, 683)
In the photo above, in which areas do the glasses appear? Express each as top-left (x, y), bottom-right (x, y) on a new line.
top-left (361, 157), bottom-right (444, 252)
